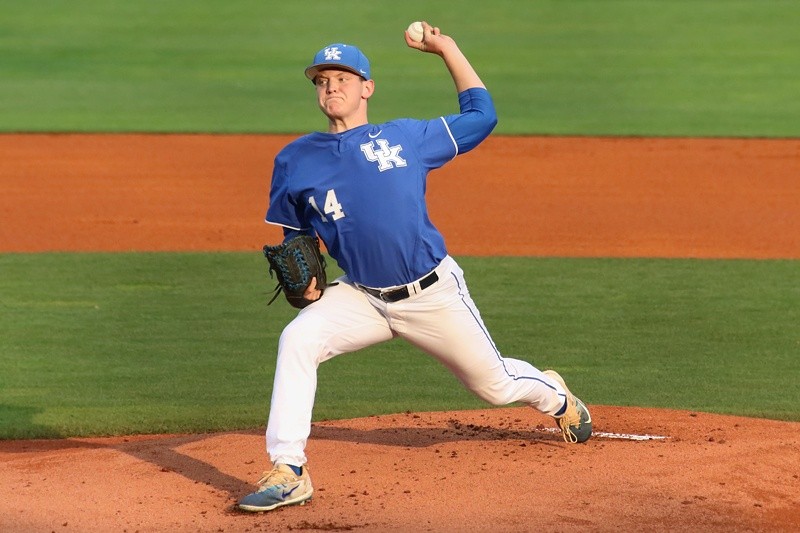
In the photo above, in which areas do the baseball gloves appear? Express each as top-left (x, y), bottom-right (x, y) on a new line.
top-left (262, 235), bottom-right (340, 309)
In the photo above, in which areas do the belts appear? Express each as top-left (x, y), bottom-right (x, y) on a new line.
top-left (352, 271), bottom-right (439, 303)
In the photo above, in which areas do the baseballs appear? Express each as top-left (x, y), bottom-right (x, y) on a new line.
top-left (408, 21), bottom-right (431, 42)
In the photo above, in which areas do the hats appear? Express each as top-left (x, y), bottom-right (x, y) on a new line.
top-left (305, 43), bottom-right (371, 82)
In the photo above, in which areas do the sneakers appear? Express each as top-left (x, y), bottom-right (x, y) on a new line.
top-left (238, 462), bottom-right (313, 511)
top-left (541, 369), bottom-right (592, 443)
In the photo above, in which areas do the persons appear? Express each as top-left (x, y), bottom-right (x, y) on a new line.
top-left (236, 22), bottom-right (591, 510)
